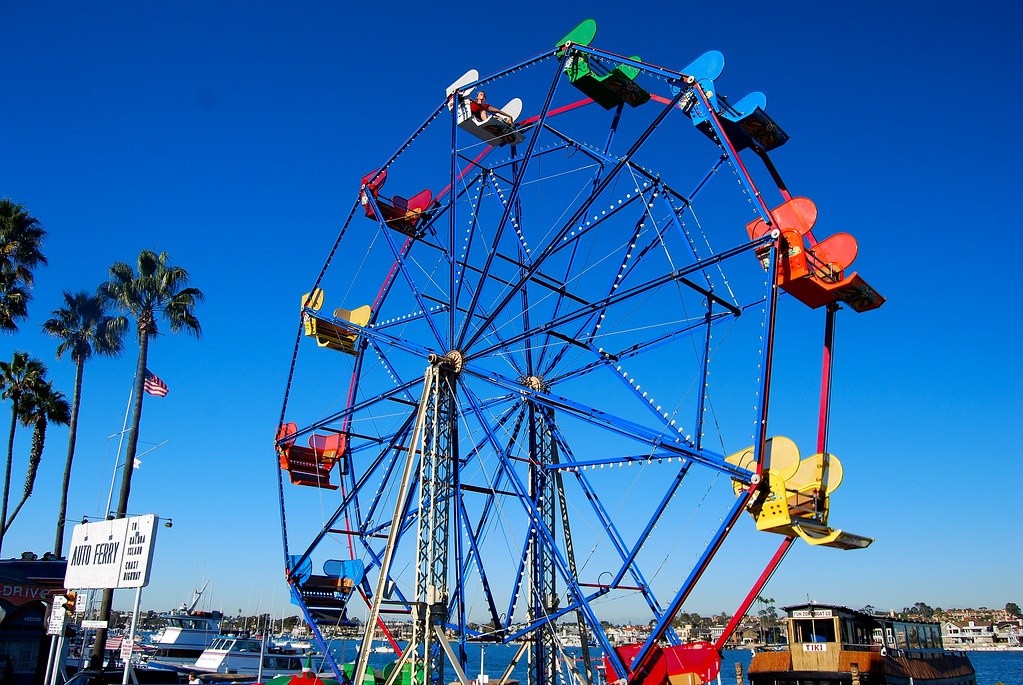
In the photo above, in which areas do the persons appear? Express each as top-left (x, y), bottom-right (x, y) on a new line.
top-left (188, 672), bottom-right (203, 684)
top-left (470, 91), bottom-right (513, 125)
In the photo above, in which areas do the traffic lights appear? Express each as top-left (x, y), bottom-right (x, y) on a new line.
top-left (62, 592), bottom-right (77, 616)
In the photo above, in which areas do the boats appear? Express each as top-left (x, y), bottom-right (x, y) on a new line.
top-left (147, 636), bottom-right (344, 685)
top-left (135, 615), bottom-right (223, 657)
top-left (291, 641), bottom-right (315, 649)
top-left (355, 645), bottom-right (375, 653)
top-left (375, 642), bottom-right (395, 652)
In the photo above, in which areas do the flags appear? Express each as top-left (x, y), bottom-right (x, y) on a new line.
top-left (144, 368), bottom-right (170, 397)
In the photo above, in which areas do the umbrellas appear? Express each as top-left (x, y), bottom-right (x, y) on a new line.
top-left (92, 634), bottom-right (156, 668)
